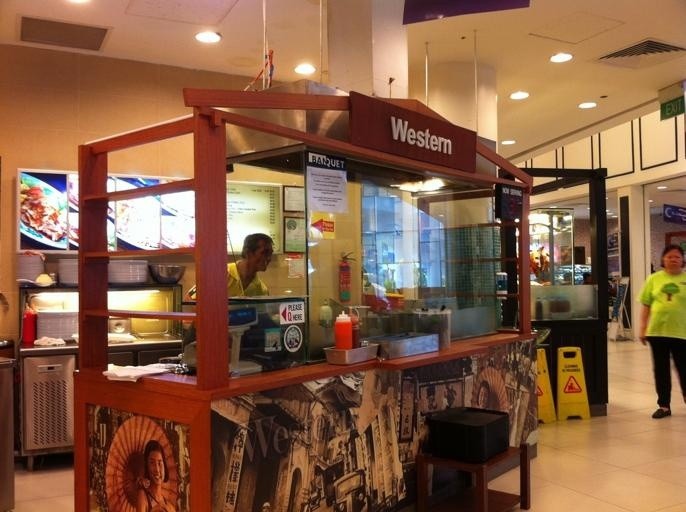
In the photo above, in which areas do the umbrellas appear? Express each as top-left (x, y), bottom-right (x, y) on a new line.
top-left (104, 414), bottom-right (180, 512)
top-left (471, 366), bottom-right (508, 412)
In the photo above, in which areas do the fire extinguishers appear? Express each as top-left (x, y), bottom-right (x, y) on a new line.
top-left (19, 305), bottom-right (37, 347)
top-left (339, 251), bottom-right (358, 303)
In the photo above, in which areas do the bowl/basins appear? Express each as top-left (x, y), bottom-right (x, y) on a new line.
top-left (148, 264), bottom-right (185, 285)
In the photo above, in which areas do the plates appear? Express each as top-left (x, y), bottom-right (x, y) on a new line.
top-left (18, 255), bottom-right (45, 285)
top-left (116, 177), bottom-right (160, 251)
top-left (108, 259), bottom-right (148, 284)
top-left (58, 259), bottom-right (79, 287)
top-left (20, 172), bottom-right (67, 248)
top-left (68, 212), bottom-right (116, 251)
top-left (36, 310), bottom-right (78, 343)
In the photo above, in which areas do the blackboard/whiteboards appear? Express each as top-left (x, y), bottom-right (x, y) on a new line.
top-left (612, 283), bottom-right (626, 317)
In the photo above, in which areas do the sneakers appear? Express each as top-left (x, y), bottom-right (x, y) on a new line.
top-left (652, 408), bottom-right (671, 419)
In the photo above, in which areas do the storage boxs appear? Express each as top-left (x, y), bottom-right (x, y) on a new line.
top-left (424, 407), bottom-right (510, 461)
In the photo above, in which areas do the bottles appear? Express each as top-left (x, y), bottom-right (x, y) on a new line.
top-left (334, 310), bottom-right (352, 350)
top-left (349, 307), bottom-right (360, 349)
top-left (535, 297), bottom-right (542, 320)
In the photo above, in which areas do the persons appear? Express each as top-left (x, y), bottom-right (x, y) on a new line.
top-left (181, 233), bottom-right (275, 347)
top-left (134, 439), bottom-right (175, 512)
top-left (560, 273), bottom-right (573, 285)
top-left (476, 380), bottom-right (489, 409)
top-left (634, 243), bottom-right (686, 419)
top-left (581, 273), bottom-right (591, 285)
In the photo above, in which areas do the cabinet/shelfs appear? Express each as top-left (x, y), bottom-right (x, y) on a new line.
top-left (417, 445), bottom-right (528, 511)
